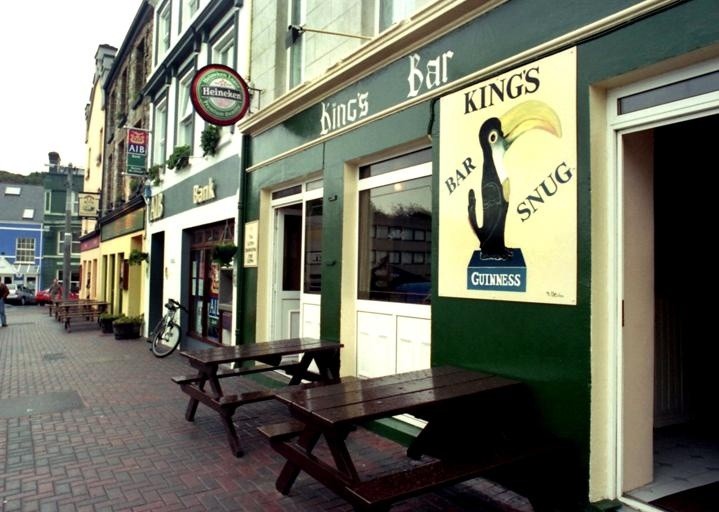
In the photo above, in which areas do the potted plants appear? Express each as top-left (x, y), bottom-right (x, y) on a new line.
top-left (99, 312), bottom-right (146, 340)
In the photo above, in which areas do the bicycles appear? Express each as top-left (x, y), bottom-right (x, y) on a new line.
top-left (146, 299), bottom-right (189, 357)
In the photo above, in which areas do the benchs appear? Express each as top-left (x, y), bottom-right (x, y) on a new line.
top-left (259, 420), bottom-right (305, 440)
top-left (48, 305), bottom-right (109, 333)
top-left (171, 361), bottom-right (363, 458)
top-left (343, 437), bottom-right (529, 509)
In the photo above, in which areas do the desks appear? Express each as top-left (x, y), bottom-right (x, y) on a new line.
top-left (270, 365), bottom-right (524, 511)
top-left (51, 298), bottom-right (112, 332)
top-left (176, 338), bottom-right (344, 458)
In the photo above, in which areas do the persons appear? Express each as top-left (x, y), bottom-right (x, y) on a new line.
top-left (0, 277), bottom-right (9, 327)
top-left (47, 279), bottom-right (63, 306)
top-left (87, 272), bottom-right (90, 299)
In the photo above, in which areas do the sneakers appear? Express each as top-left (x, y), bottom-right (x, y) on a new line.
top-left (2, 324), bottom-right (8, 327)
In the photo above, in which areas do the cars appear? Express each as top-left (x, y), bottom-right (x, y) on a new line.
top-left (6, 284), bottom-right (79, 307)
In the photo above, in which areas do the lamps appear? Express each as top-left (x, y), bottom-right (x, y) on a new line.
top-left (284, 24), bottom-right (372, 48)
